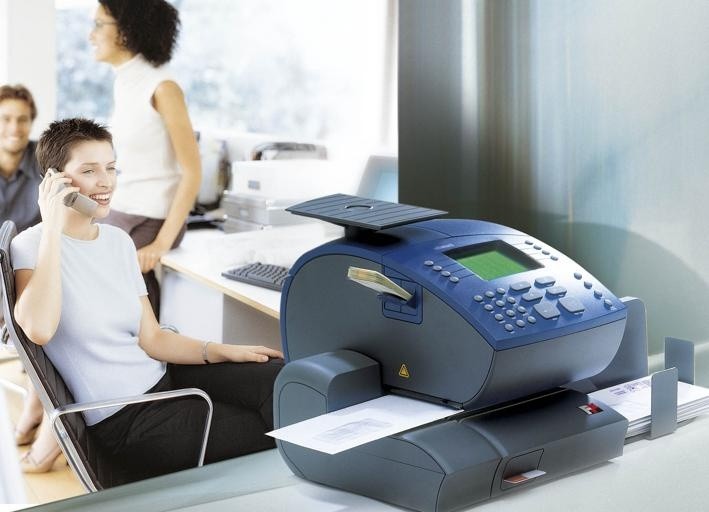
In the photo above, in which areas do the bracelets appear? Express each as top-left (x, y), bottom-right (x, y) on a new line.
top-left (202, 339), bottom-right (215, 364)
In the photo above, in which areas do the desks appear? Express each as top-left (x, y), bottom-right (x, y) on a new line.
top-left (157, 225), bottom-right (344, 353)
top-left (10, 343), bottom-right (709, 511)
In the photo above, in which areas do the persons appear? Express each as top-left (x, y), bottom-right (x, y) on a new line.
top-left (13, 0), bottom-right (203, 476)
top-left (8, 114), bottom-right (284, 479)
top-left (1, 86), bottom-right (50, 232)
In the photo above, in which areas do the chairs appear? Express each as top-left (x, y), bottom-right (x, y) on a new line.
top-left (0, 220), bottom-right (214, 493)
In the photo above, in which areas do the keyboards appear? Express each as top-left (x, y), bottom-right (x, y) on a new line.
top-left (221, 260), bottom-right (290, 292)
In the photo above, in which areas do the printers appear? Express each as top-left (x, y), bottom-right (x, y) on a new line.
top-left (271, 193), bottom-right (629, 504)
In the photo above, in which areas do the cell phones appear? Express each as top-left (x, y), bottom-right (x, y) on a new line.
top-left (39, 169), bottom-right (99, 220)
